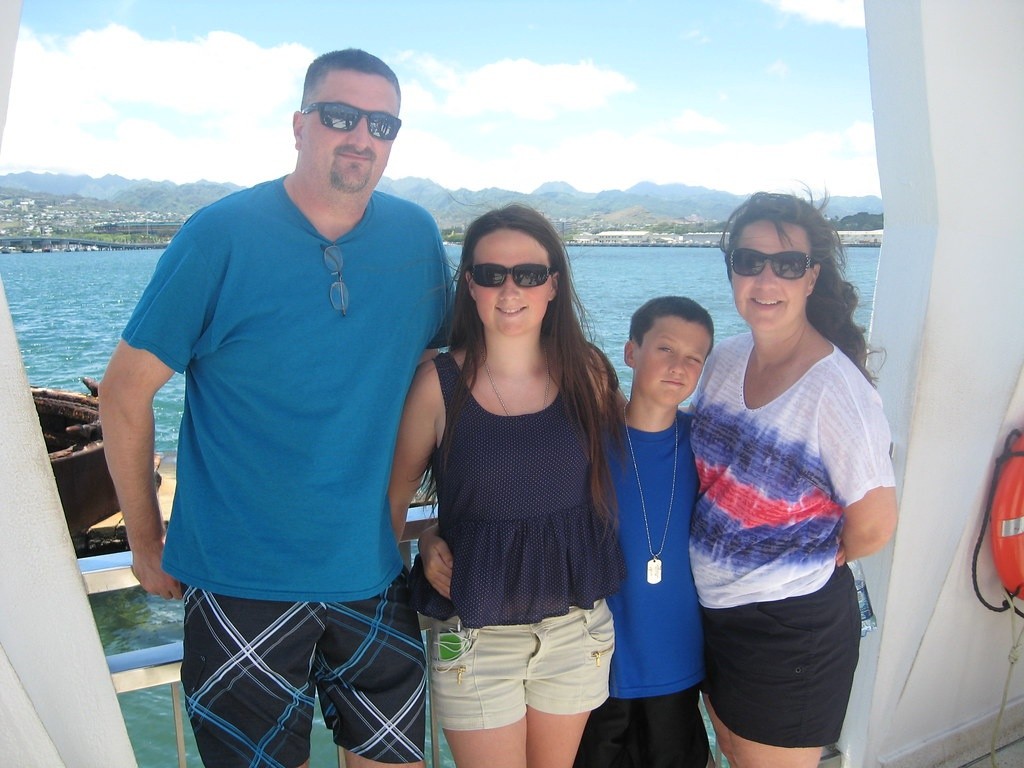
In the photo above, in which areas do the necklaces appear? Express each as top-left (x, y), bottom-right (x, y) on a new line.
top-left (624, 401), bottom-right (678, 585)
top-left (479, 339), bottom-right (549, 416)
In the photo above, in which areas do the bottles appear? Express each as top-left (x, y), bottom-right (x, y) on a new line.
top-left (846, 559), bottom-right (877, 637)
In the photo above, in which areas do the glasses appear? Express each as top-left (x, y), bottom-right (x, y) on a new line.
top-left (300, 102), bottom-right (402, 141)
top-left (468, 263), bottom-right (555, 287)
top-left (729, 247), bottom-right (818, 280)
top-left (321, 242), bottom-right (350, 318)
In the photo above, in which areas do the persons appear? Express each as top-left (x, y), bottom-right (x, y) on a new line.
top-left (676, 193), bottom-right (899, 768)
top-left (100, 50), bottom-right (455, 768)
top-left (418, 298), bottom-right (845, 768)
top-left (389, 207), bottom-right (630, 768)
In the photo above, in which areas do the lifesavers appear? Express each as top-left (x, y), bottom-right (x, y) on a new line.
top-left (990, 430), bottom-right (1024, 599)
